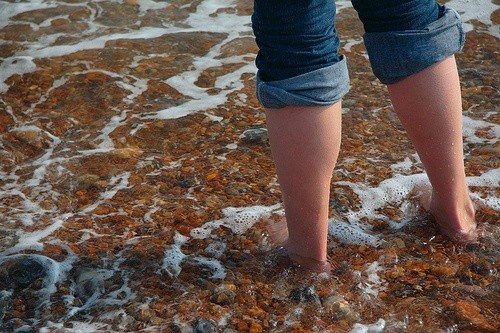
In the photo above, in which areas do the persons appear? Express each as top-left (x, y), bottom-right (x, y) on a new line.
top-left (250, 0), bottom-right (479, 280)
